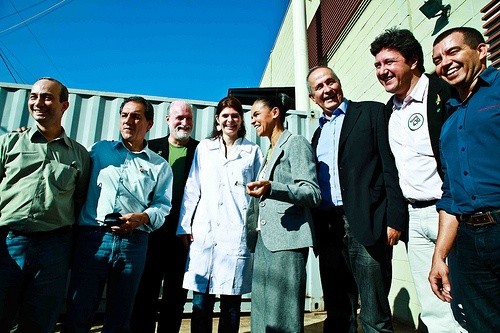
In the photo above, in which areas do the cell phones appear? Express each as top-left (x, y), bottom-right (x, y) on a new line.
top-left (105, 219), bottom-right (121, 227)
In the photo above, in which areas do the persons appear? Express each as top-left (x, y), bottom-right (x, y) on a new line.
top-left (11, 95), bottom-right (174, 333)
top-left (242, 93), bottom-right (323, 332)
top-left (175, 96), bottom-right (266, 333)
top-left (305, 64), bottom-right (409, 332)
top-left (427, 25), bottom-right (499, 333)
top-left (0, 76), bottom-right (93, 333)
top-left (130, 98), bottom-right (202, 333)
top-left (370, 27), bottom-right (468, 332)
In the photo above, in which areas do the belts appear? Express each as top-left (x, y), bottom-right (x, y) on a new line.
top-left (457, 210), bottom-right (500, 227)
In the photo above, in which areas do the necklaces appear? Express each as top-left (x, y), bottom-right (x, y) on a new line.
top-left (267, 146), bottom-right (277, 161)
